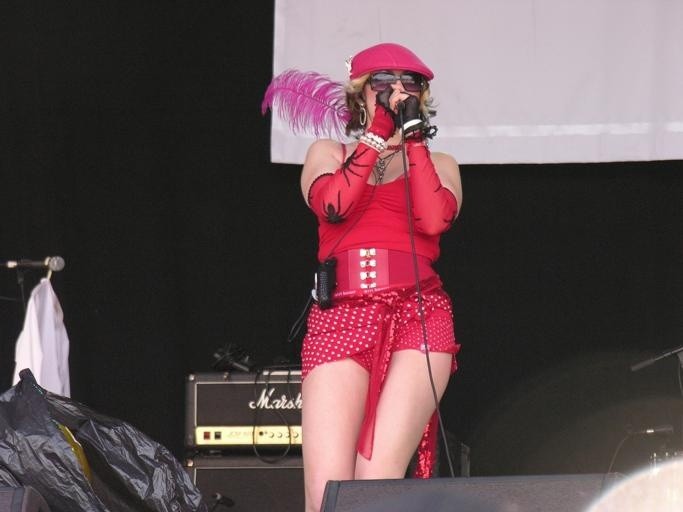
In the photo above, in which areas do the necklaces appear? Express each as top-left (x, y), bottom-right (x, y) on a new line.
top-left (371, 139), bottom-right (403, 186)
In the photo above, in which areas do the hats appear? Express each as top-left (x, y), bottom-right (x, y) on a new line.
top-left (350, 44), bottom-right (434, 80)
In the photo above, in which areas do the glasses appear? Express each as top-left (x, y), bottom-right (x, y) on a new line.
top-left (365, 72), bottom-right (423, 92)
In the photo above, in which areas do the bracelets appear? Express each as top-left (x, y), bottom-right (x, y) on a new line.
top-left (358, 131), bottom-right (389, 153)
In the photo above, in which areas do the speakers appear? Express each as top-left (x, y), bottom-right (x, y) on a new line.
top-left (319, 472), bottom-right (626, 511)
top-left (186, 458), bottom-right (306, 512)
top-left (184, 370), bottom-right (303, 447)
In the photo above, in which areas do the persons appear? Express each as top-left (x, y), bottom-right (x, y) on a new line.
top-left (296, 41), bottom-right (467, 511)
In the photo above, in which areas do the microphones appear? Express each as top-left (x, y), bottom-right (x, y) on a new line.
top-left (7, 257), bottom-right (65, 272)
